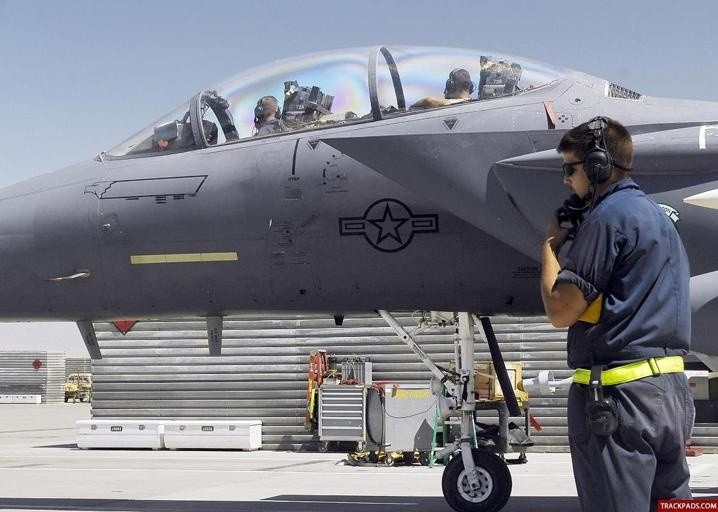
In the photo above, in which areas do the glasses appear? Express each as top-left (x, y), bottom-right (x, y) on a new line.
top-left (562, 161), bottom-right (583, 176)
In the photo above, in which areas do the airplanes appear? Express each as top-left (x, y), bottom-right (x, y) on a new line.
top-left (0, 42), bottom-right (718, 512)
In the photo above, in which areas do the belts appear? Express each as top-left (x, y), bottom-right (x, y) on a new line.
top-left (573, 356), bottom-right (685, 387)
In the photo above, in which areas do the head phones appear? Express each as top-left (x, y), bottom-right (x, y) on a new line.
top-left (583, 117), bottom-right (612, 184)
top-left (447, 68), bottom-right (475, 94)
top-left (254, 95), bottom-right (281, 117)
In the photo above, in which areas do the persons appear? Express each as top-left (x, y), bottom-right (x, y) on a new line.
top-left (541, 115), bottom-right (692, 512)
top-left (252, 95), bottom-right (282, 135)
top-left (409, 67), bottom-right (473, 111)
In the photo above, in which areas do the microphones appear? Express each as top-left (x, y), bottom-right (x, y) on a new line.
top-left (563, 183), bottom-right (598, 213)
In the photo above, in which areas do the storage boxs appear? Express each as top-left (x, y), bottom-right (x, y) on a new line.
top-left (449, 360), bottom-right (522, 401)
